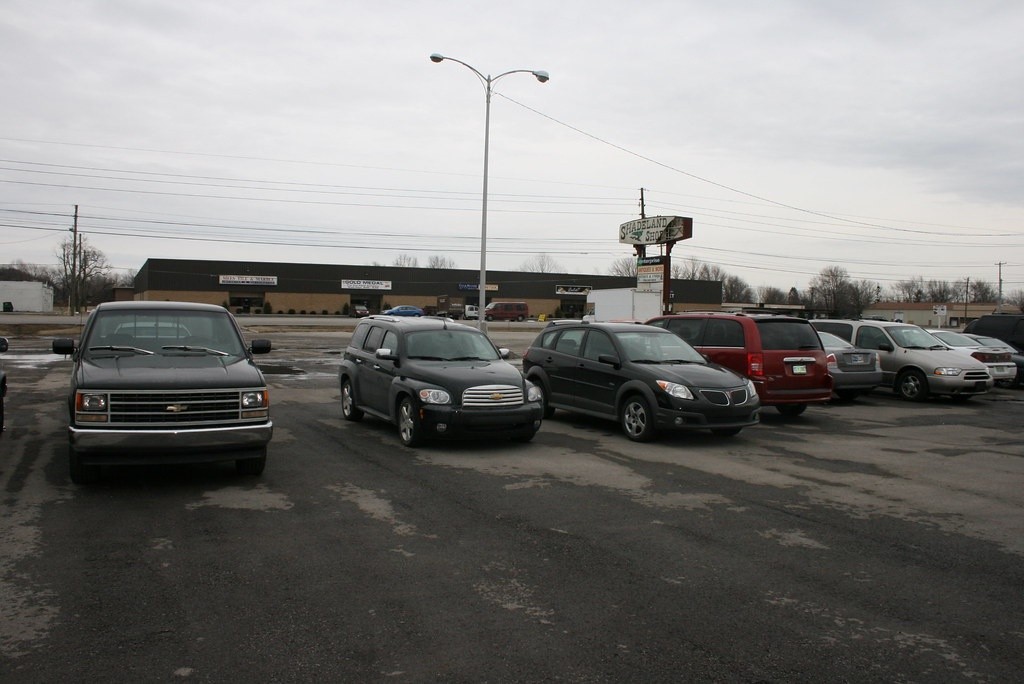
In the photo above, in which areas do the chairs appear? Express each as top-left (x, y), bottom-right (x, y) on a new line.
top-left (104, 334), bottom-right (136, 347)
top-left (182, 336), bottom-right (209, 348)
top-left (558, 339), bottom-right (578, 355)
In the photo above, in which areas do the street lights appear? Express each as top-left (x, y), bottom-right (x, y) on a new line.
top-left (429, 53), bottom-right (550, 337)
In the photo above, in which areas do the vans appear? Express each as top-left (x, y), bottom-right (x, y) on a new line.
top-left (484, 302), bottom-right (529, 322)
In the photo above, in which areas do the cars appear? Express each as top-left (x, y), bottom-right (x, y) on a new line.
top-left (381, 305), bottom-right (424, 317)
top-left (349, 305), bottom-right (370, 318)
top-left (817, 331), bottom-right (883, 403)
top-left (113, 320), bottom-right (194, 340)
top-left (901, 313), bottom-right (1024, 390)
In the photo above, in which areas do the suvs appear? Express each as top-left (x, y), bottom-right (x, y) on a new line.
top-left (633, 310), bottom-right (837, 418)
top-left (51, 299), bottom-right (275, 479)
top-left (521, 317), bottom-right (761, 442)
top-left (807, 317), bottom-right (995, 402)
top-left (338, 313), bottom-right (546, 449)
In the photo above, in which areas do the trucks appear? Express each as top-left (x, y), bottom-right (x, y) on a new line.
top-left (448, 303), bottom-right (479, 319)
